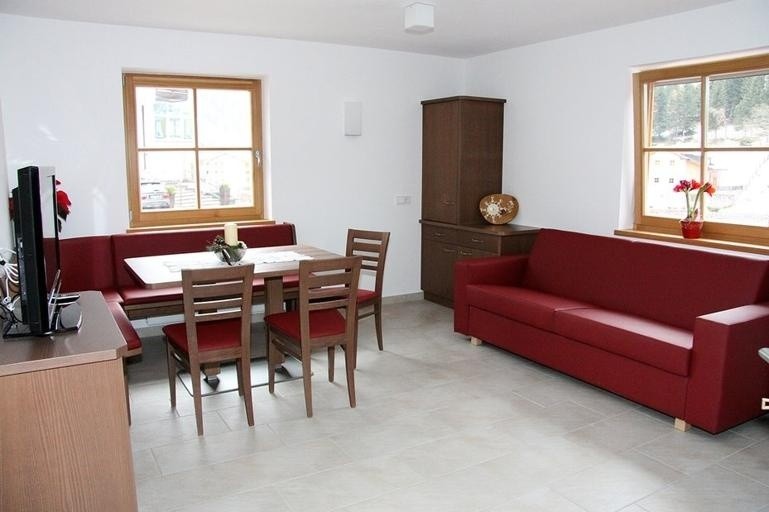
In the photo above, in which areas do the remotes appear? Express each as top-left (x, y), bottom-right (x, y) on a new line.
top-left (55, 294), bottom-right (79, 303)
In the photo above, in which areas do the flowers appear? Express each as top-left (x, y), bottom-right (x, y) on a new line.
top-left (675, 179), bottom-right (715, 220)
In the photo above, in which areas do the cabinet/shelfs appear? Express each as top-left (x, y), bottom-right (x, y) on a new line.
top-left (418, 219), bottom-right (541, 312)
top-left (421, 96), bottom-right (507, 227)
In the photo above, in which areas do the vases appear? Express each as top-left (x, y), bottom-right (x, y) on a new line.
top-left (680, 219), bottom-right (703, 240)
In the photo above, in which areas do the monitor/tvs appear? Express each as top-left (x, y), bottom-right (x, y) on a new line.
top-left (2, 165), bottom-right (83, 338)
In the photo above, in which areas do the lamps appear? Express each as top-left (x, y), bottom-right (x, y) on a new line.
top-left (403, 1), bottom-right (438, 35)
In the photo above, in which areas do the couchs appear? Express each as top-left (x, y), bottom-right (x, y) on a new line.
top-left (59, 235), bottom-right (122, 303)
top-left (454, 228), bottom-right (719, 434)
top-left (111, 222), bottom-right (322, 319)
top-left (0, 290), bottom-right (141, 510)
top-left (109, 302), bottom-right (143, 358)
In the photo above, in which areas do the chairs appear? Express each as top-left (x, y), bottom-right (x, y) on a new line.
top-left (163, 263), bottom-right (256, 436)
top-left (317, 229), bottom-right (391, 368)
top-left (264, 256), bottom-right (362, 418)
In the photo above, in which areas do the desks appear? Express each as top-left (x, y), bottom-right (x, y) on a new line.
top-left (124, 244), bottom-right (350, 376)
